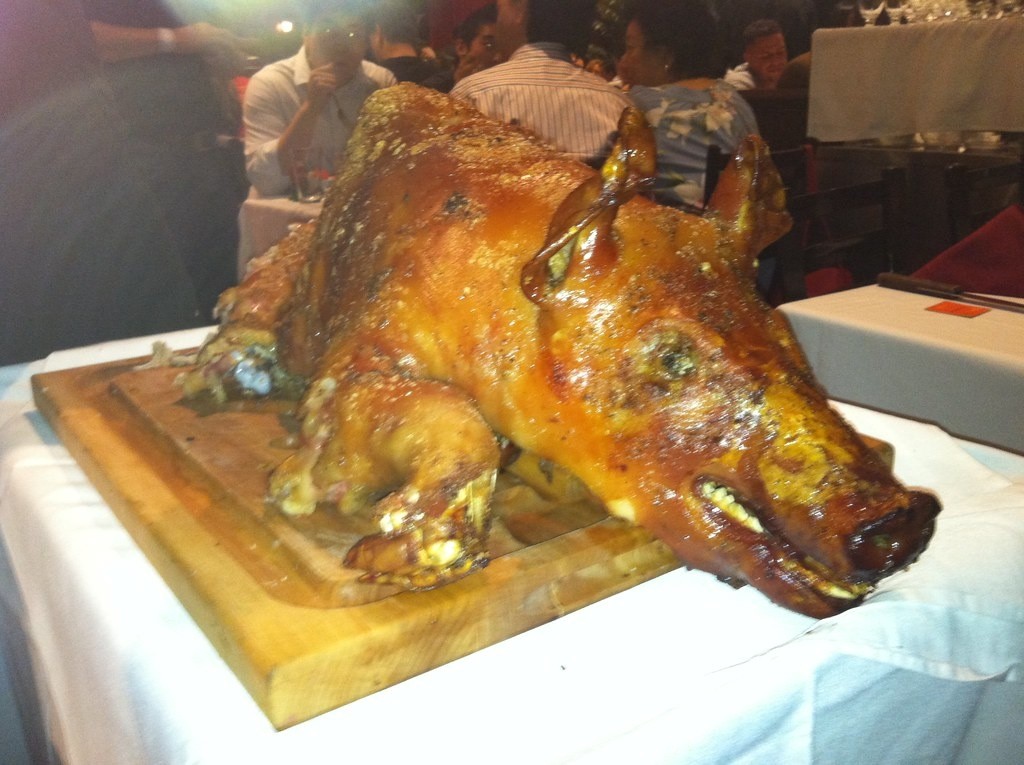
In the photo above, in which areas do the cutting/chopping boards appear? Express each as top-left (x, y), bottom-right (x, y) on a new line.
top-left (29, 331), bottom-right (682, 731)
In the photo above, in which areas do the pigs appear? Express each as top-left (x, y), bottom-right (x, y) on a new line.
top-left (175, 82), bottom-right (940, 621)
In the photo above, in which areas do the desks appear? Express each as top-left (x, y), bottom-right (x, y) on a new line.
top-left (238, 194), bottom-right (323, 288)
top-left (0, 323), bottom-right (1024, 765)
top-left (774, 280), bottom-right (1023, 455)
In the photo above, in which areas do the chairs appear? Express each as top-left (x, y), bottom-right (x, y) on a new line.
top-left (705, 87), bottom-right (1024, 304)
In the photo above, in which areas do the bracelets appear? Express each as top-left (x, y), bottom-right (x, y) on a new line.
top-left (156, 27), bottom-right (178, 55)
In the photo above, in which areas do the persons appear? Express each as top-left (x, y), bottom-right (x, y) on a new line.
top-left (0, 0), bottom-right (893, 362)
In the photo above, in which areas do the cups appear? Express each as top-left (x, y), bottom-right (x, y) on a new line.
top-left (287, 145), bottom-right (323, 203)
top-left (857, 0), bottom-right (1023, 27)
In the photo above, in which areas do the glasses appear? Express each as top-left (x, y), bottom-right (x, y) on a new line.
top-left (317, 23), bottom-right (362, 44)
